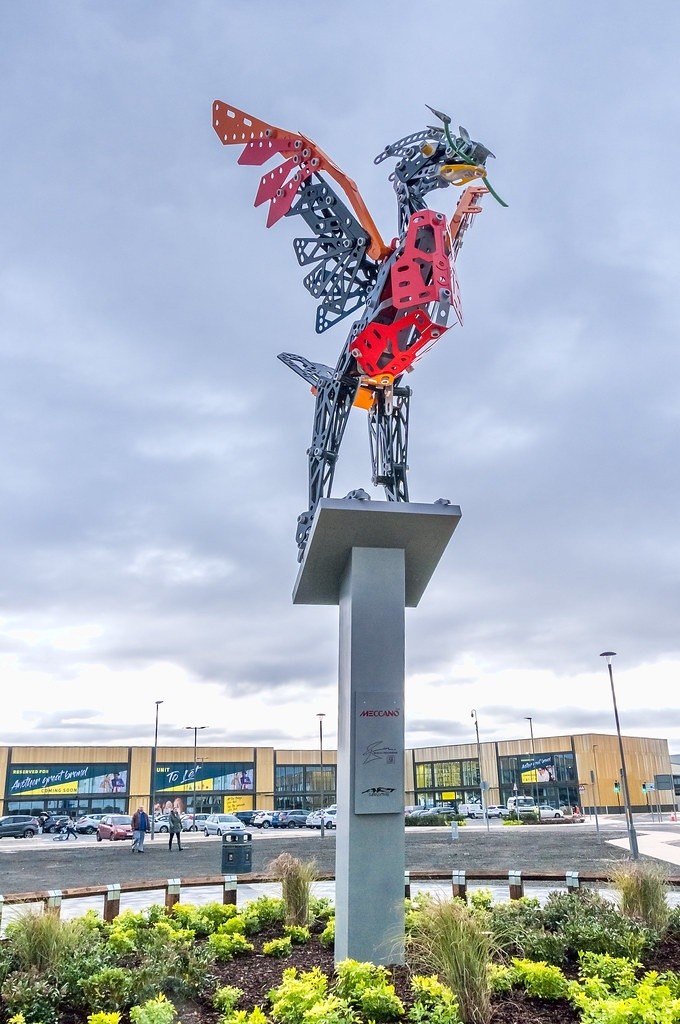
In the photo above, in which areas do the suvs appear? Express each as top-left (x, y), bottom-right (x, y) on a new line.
top-left (405, 806), bottom-right (455, 817)
top-left (0, 814), bottom-right (41, 839)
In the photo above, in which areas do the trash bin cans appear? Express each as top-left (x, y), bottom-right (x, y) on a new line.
top-left (221, 830), bottom-right (253, 875)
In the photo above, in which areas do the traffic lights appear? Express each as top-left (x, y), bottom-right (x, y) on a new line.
top-left (614, 782), bottom-right (618, 791)
top-left (642, 783), bottom-right (646, 792)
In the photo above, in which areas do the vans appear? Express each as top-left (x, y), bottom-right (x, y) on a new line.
top-left (458, 804), bottom-right (483, 819)
top-left (507, 795), bottom-right (536, 817)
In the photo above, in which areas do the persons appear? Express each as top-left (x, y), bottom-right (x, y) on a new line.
top-left (537, 767), bottom-right (549, 782)
top-left (168, 807), bottom-right (183, 851)
top-left (99, 773), bottom-right (125, 793)
top-left (131, 806), bottom-right (151, 853)
top-left (231, 771), bottom-right (252, 790)
top-left (65, 816), bottom-right (78, 840)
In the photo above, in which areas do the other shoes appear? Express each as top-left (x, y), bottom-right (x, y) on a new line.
top-left (179, 848), bottom-right (183, 851)
top-left (138, 850), bottom-right (144, 853)
top-left (132, 847), bottom-right (134, 852)
top-left (169, 849), bottom-right (172, 852)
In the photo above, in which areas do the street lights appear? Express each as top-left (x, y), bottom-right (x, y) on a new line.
top-left (317, 713), bottom-right (324, 839)
top-left (599, 651), bottom-right (640, 860)
top-left (186, 727), bottom-right (206, 832)
top-left (151, 700), bottom-right (164, 840)
top-left (593, 744), bottom-right (599, 832)
top-left (526, 717), bottom-right (541, 821)
top-left (471, 708), bottom-right (486, 820)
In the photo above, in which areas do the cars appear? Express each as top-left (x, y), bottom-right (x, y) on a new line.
top-left (536, 805), bottom-right (564, 818)
top-left (488, 805), bottom-right (509, 819)
top-left (97, 815), bottom-right (134, 842)
top-left (204, 814), bottom-right (246, 837)
top-left (231, 804), bottom-right (337, 830)
top-left (146, 813), bottom-right (210, 833)
top-left (38, 810), bottom-right (108, 835)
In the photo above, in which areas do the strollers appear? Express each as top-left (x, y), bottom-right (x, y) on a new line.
top-left (53, 826), bottom-right (67, 841)
